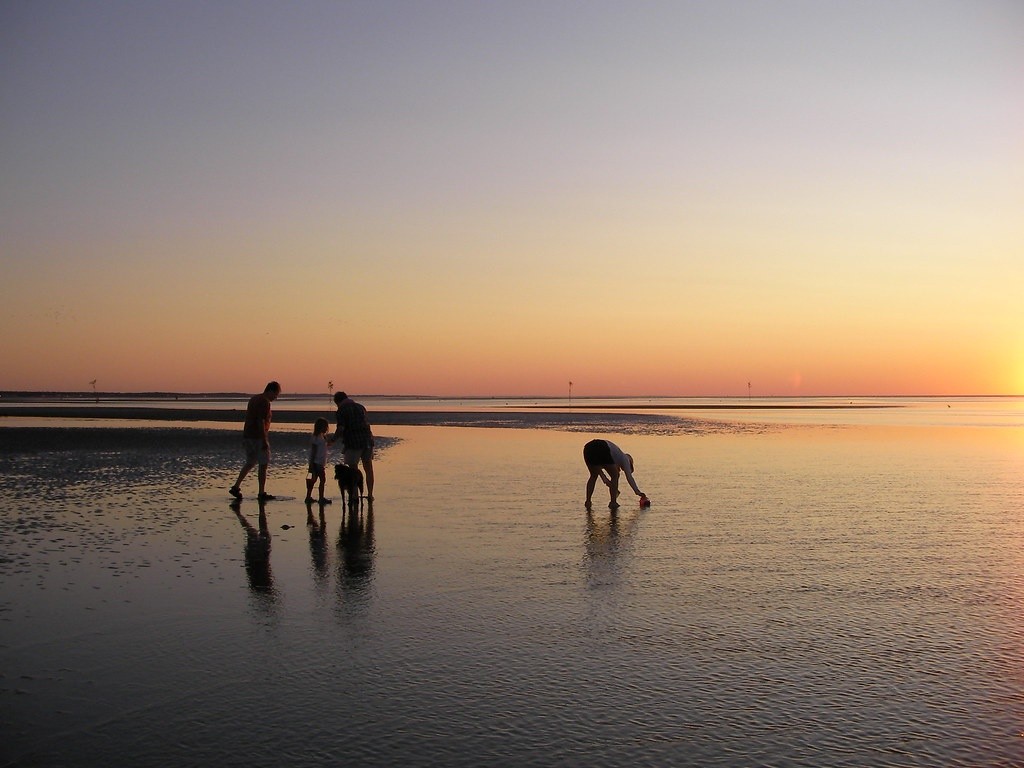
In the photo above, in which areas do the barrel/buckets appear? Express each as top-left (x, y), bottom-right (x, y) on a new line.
top-left (305, 472), bottom-right (316, 488)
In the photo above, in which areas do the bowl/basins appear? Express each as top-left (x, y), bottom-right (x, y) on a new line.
top-left (640, 499), bottom-right (650, 506)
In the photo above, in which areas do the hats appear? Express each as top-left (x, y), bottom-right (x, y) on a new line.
top-left (626, 454), bottom-right (634, 473)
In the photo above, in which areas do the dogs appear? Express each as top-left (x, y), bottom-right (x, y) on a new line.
top-left (334, 464), bottom-right (364, 505)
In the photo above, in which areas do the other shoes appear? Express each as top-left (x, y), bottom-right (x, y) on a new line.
top-left (318, 498), bottom-right (332, 503)
top-left (305, 497), bottom-right (316, 503)
top-left (258, 493), bottom-right (272, 499)
top-left (228, 486), bottom-right (242, 498)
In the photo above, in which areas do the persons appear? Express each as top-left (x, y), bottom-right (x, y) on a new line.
top-left (583, 438), bottom-right (645, 507)
top-left (327, 391), bottom-right (375, 497)
top-left (305, 417), bottom-right (332, 504)
top-left (229, 381), bottom-right (281, 500)
